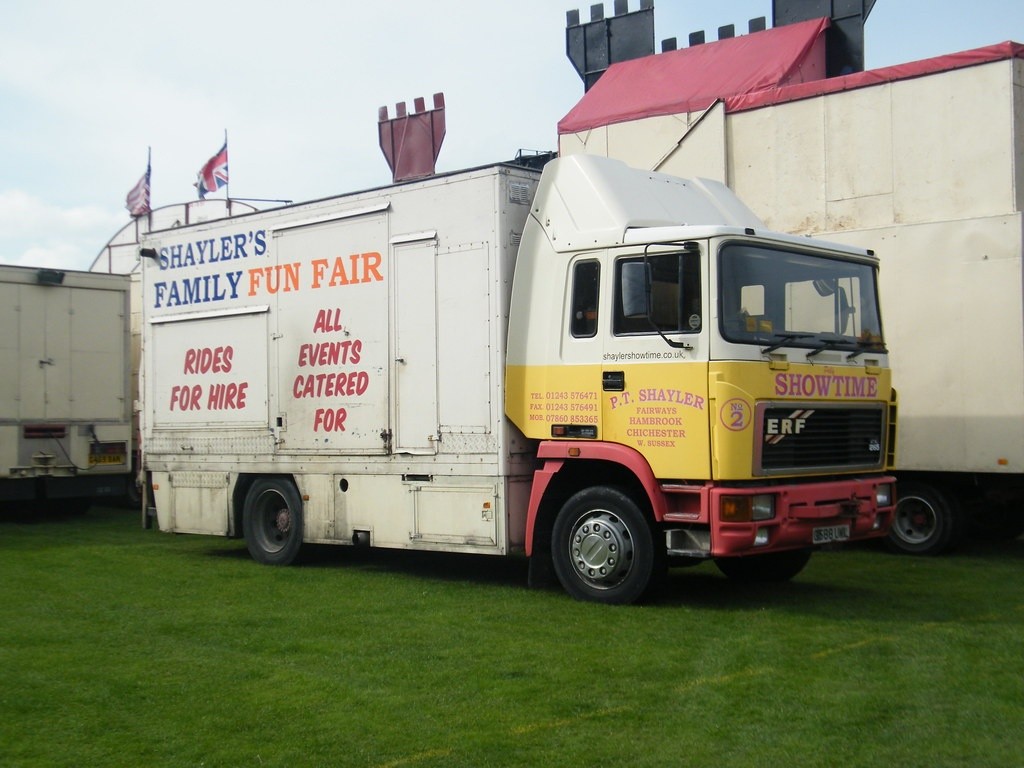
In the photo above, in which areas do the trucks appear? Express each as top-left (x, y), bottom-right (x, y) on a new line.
top-left (133, 153), bottom-right (901, 607)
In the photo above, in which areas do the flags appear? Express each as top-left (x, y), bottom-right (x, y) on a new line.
top-left (124, 164), bottom-right (152, 218)
top-left (192, 142), bottom-right (228, 200)
